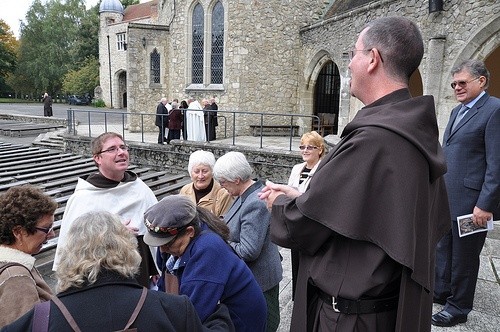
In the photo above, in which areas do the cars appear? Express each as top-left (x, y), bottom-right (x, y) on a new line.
top-left (67, 94), bottom-right (88, 106)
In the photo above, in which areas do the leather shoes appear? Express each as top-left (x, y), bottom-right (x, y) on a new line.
top-left (431, 309), bottom-right (467, 327)
top-left (432, 297), bottom-right (445, 305)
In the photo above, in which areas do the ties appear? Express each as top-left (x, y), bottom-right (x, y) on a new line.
top-left (452, 106), bottom-right (469, 131)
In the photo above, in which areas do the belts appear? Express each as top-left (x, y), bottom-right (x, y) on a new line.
top-left (308, 279), bottom-right (400, 315)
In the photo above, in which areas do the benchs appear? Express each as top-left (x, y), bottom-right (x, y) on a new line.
top-left (250, 125), bottom-right (299, 136)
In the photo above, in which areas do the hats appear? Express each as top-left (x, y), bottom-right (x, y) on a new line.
top-left (142, 194), bottom-right (197, 246)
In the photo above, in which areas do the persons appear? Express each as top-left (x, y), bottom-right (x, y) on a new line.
top-left (213, 152), bottom-right (283, 332)
top-left (155, 97), bottom-right (218, 145)
top-left (143, 195), bottom-right (267, 332)
top-left (0, 210), bottom-right (203, 332)
top-left (179, 150), bottom-right (234, 217)
top-left (0, 184), bottom-right (57, 327)
top-left (52, 131), bottom-right (163, 288)
top-left (42, 92), bottom-right (52, 117)
top-left (257, 17), bottom-right (453, 332)
top-left (289, 131), bottom-right (325, 193)
top-left (432, 61), bottom-right (500, 327)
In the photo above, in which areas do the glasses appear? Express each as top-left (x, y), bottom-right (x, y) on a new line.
top-left (32, 224), bottom-right (53, 234)
top-left (452, 76), bottom-right (483, 89)
top-left (97, 145), bottom-right (130, 155)
top-left (348, 49), bottom-right (383, 63)
top-left (299, 145), bottom-right (317, 150)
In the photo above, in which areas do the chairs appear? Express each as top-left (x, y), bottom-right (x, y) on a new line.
top-left (312, 113), bottom-right (335, 137)
